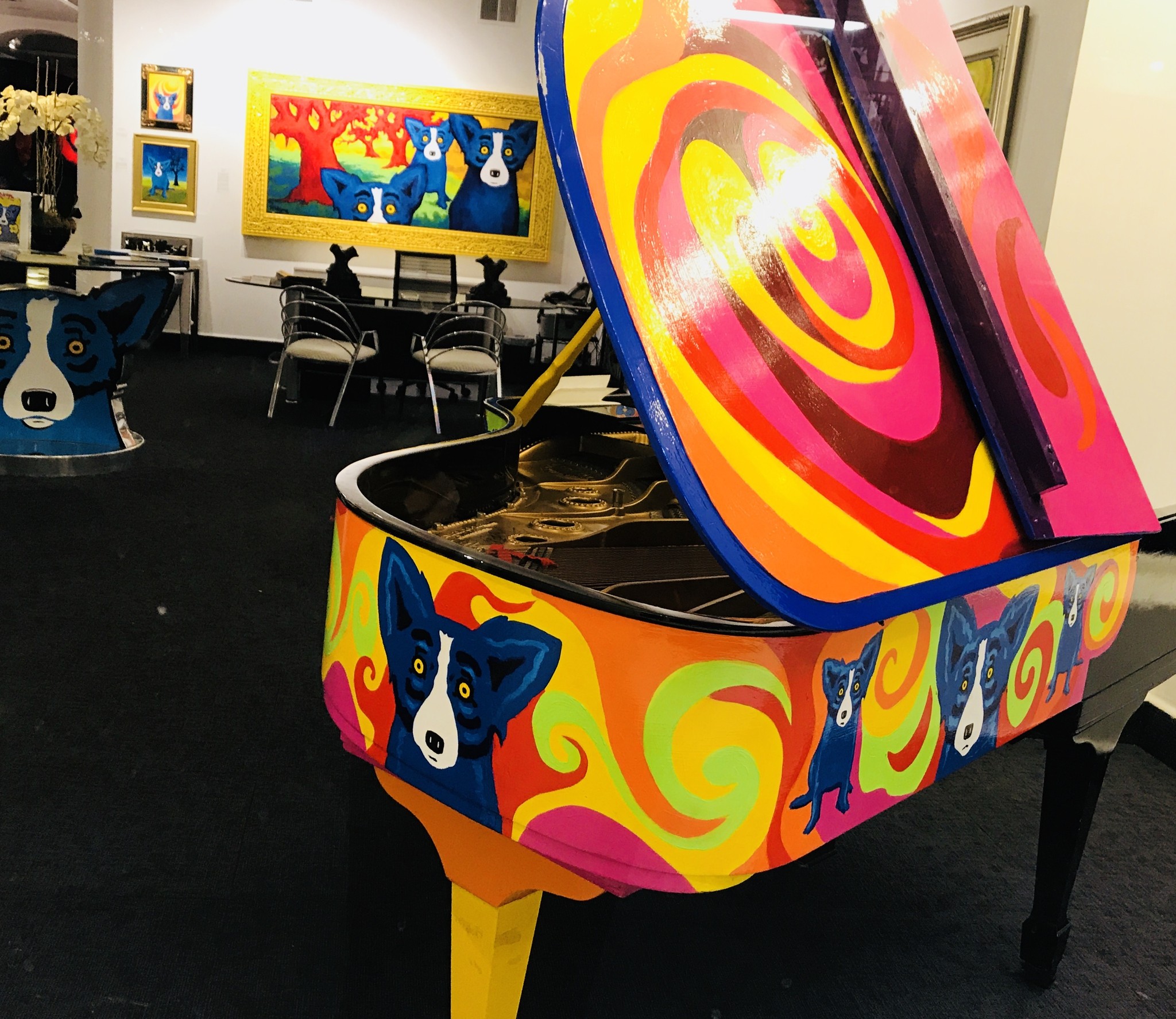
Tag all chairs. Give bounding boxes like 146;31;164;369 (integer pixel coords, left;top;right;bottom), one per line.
267;284;379;426
394;249;472;399
410;300;506;435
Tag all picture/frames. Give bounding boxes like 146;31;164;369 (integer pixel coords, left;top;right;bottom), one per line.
121;232;192;258
950;4;1030;162
241;71;557;262
141;64;193;133
0;189;31;252
132;133;199;217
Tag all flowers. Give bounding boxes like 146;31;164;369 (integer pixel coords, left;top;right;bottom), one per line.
0;57;109;214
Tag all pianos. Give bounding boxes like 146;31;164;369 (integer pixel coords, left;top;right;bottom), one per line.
321;0;1162;1019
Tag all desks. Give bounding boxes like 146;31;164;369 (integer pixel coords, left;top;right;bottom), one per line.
226;275;556;418
0;249;199;480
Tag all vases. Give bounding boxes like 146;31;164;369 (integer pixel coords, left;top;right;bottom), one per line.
32;193;79;256
